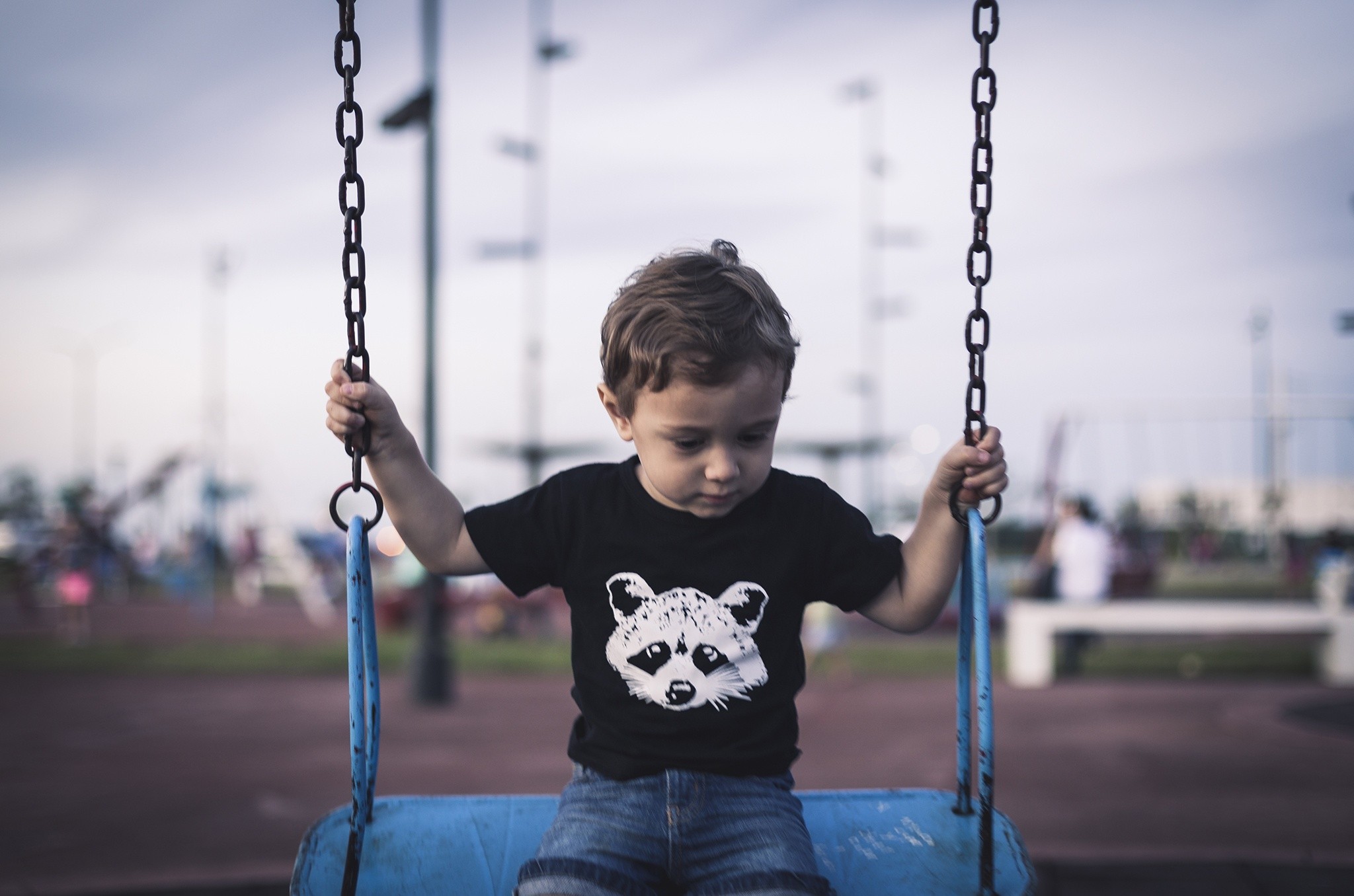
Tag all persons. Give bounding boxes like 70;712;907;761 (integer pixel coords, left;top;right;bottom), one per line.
1010;490;1150;676
325;238;1010;896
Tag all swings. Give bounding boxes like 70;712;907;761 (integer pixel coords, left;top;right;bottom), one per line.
287;1;1042;895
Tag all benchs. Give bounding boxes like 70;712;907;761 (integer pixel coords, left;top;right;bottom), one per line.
999;595;1353;691
